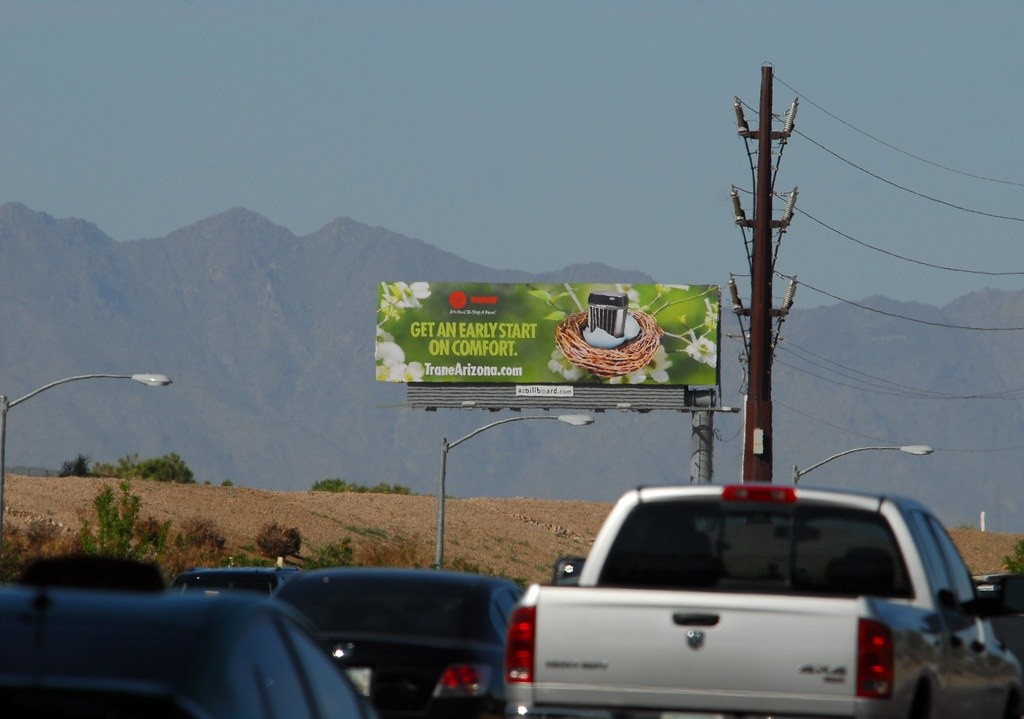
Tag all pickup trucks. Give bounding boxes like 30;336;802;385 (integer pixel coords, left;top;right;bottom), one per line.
497;484;1024;719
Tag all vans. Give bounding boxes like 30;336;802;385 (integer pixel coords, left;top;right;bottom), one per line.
172;565;299;601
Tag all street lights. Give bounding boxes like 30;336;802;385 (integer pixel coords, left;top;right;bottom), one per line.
0;372;176;580
433;413;596;576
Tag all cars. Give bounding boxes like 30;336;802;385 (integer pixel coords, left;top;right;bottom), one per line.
263;569;538;719
0;583;383;719
